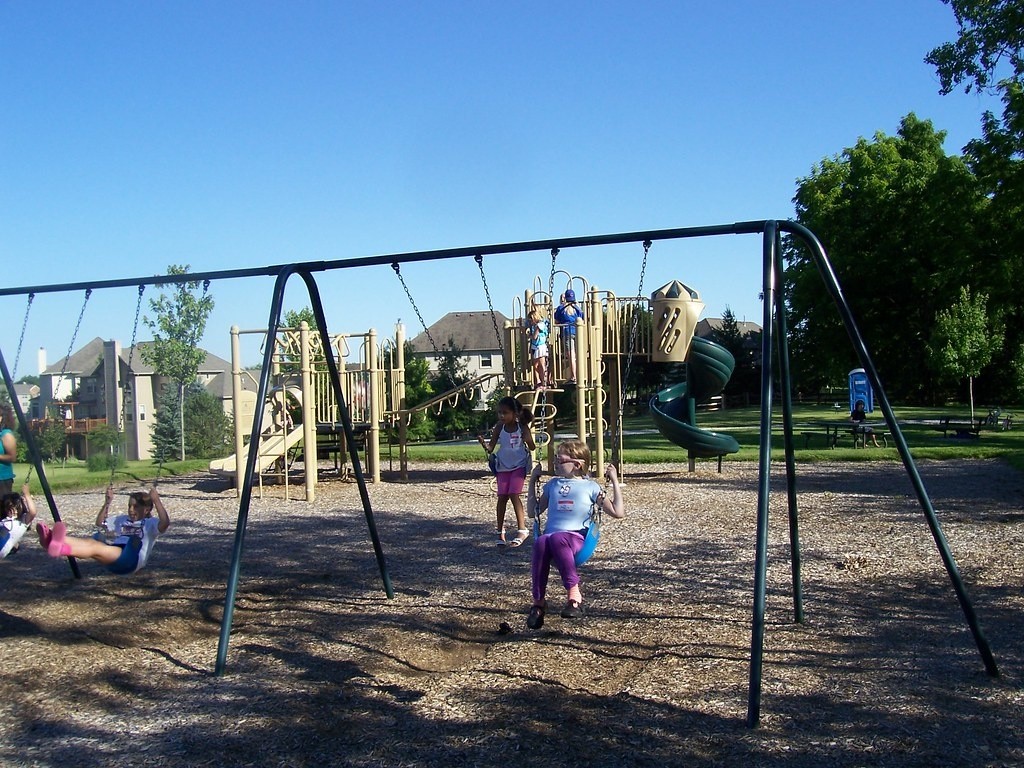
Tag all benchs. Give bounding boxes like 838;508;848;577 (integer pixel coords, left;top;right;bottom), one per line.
845;428;891;448
801;432;846;450
984;414;1013;430
931;415;988;439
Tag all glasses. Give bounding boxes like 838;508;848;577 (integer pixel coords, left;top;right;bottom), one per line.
552;455;583;470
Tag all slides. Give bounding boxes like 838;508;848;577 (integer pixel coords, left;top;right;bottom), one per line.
206;421;307;483
645;332;742;459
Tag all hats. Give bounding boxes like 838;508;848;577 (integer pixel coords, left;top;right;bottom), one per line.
565;290;575;302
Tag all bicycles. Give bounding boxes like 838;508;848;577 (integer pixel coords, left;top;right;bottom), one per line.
1001;413;1015;431
985;408;1002;428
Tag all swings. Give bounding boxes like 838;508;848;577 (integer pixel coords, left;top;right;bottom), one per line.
86;279;211;579
0;283;94;556
528;237;655;574
389;253;534;481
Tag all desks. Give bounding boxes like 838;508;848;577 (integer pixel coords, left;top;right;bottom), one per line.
818;421;887;449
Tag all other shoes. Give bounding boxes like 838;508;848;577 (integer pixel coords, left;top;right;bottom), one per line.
873;443;880;447
527;598;546;629
534;382;546;391
48;521;66;558
35;521;52;550
8;546;20;554
565;378;577;385
561;599;585;618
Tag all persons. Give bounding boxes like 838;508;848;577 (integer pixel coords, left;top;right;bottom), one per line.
0;404;21;554
36;487;170;576
849;399;881;448
993;406;1013;431
477;395;536;548
0;483;37;562
519;308;551;388
526;438;625;630
554;289;584;385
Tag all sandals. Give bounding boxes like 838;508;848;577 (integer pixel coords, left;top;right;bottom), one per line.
494;529;506;547
509;527;529;547
